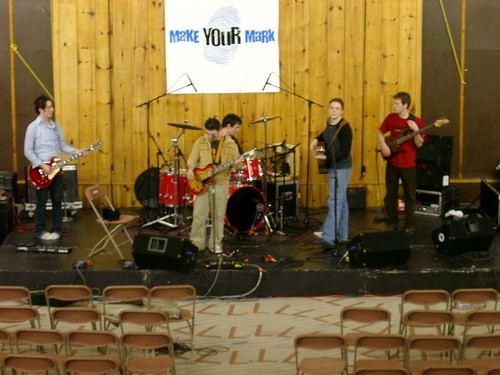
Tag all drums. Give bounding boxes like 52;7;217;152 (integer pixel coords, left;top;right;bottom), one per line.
225;184;267;233
238;152;264;181
158;166;194;207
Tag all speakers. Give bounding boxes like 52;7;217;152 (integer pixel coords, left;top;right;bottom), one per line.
415;135;453;192
131;232;199;271
432;216;495;256
346;187;366;210
25;163;77;203
347;229;411;269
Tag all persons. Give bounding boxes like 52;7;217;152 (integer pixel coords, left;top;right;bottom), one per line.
311;97;353;248
377;92;428;234
24;95;89;240
188;113;245;254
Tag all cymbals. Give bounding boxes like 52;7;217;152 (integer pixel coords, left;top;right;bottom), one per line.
168;122;203;130
249;116;281;123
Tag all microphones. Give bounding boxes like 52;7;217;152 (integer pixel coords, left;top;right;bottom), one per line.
187;74;197;92
263;73;272;91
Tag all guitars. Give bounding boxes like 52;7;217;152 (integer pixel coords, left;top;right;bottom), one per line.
187;148;257;195
380;117;450;160
27;140;103;189
314;142;329;174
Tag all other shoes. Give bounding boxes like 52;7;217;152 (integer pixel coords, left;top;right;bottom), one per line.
385;223;398;233
312;231;335;249
48;232;62;240
37;232;51;240
208;245;223;254
404;226;415;236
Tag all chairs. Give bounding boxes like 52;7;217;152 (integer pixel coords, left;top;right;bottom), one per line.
0;285;197;375
294;288;500;375
84;184;138;260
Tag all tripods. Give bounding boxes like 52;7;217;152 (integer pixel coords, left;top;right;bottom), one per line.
268;83;324;230
121;84;234;236
